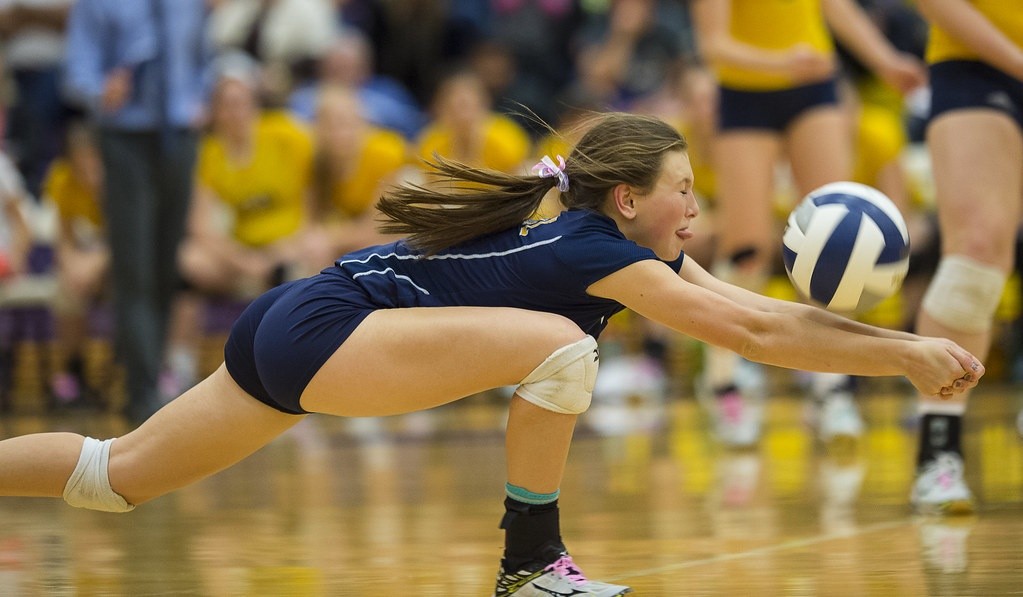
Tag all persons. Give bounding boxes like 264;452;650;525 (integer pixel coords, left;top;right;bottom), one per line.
0;97;985;597
0;0;1023;511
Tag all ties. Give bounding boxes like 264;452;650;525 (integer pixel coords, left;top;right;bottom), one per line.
145;0;170;125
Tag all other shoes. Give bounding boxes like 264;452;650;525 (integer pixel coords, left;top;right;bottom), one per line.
912;451;971;508
694;375;759;448
816;390;857;442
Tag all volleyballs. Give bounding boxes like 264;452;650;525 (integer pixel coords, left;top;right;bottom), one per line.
782;181;910;313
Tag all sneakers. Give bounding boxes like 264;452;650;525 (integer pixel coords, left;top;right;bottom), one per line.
494;542;632;596
